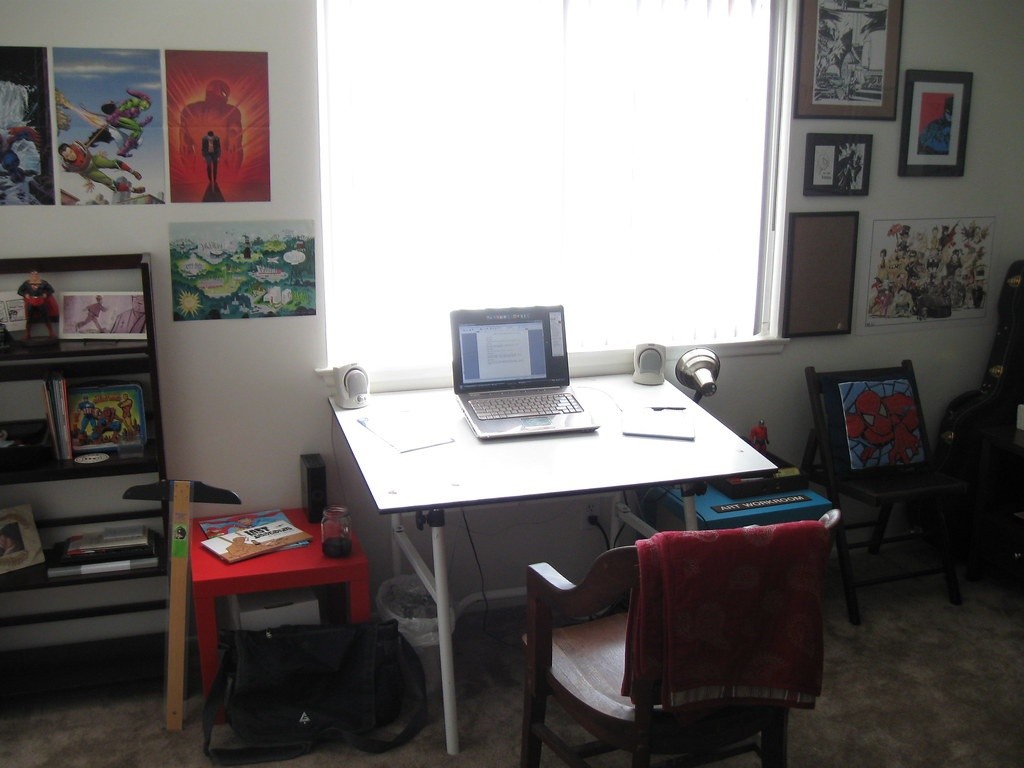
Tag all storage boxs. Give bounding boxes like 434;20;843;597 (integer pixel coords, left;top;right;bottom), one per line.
0;416;50;473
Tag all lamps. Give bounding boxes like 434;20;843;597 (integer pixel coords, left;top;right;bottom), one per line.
675;347;721;404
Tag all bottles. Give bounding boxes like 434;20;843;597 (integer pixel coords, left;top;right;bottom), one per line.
320;508;353;560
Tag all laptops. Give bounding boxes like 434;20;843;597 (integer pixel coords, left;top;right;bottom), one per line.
450;304;601;439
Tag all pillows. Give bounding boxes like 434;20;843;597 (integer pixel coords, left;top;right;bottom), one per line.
821;370;930;479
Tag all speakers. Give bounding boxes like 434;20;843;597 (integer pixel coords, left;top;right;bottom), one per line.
633;344;669;384
334;362;370;409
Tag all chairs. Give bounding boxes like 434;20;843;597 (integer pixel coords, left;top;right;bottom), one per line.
518;508;845;768
799;359;962;626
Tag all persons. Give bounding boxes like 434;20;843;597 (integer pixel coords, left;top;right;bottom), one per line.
750;419;769;451
17;270;58;340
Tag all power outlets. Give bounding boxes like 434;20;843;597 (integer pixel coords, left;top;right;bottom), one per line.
580;501;601;530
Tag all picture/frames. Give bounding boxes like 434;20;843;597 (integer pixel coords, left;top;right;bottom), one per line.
0;291;27;333
792;0;904;121
781;211;860;338
897;68;974;177
802;131;874;197
0;504;45;574
58;290;147;340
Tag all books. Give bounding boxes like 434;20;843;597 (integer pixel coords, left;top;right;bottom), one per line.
41;371;74;462
42;525;167;587
357;410;454;454
199;509;314;565
622;407;695;440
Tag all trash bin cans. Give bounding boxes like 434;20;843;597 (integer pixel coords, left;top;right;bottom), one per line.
378;575;486;701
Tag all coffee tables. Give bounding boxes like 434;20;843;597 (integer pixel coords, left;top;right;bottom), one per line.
188;504;370;725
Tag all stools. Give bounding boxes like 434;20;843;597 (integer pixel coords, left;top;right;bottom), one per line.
634;483;833;539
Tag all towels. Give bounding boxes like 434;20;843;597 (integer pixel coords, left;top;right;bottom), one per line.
620;521;829;711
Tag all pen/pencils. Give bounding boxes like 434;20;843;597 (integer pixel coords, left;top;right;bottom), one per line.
650;406;686;411
623;431;695;442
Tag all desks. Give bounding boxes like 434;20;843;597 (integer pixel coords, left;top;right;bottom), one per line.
964;423;1024;592
327;372;781;757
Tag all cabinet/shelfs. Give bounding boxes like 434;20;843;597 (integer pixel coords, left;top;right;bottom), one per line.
0;252;195;707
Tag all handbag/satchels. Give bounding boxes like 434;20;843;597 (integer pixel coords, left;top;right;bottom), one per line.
200;617;427;766
